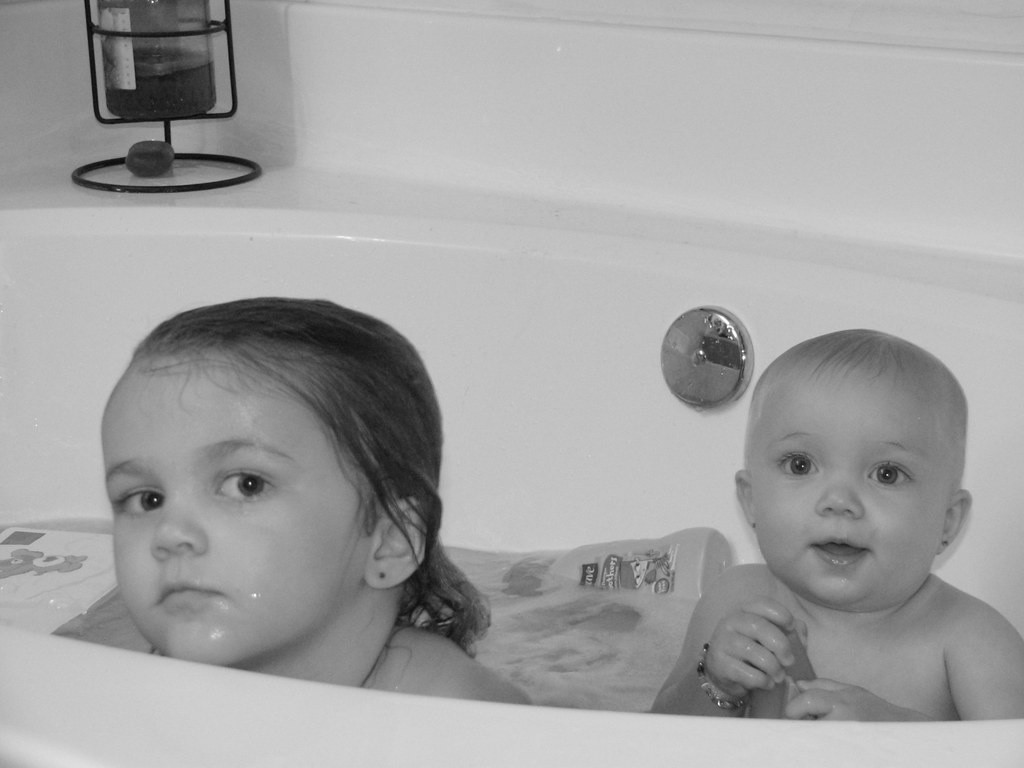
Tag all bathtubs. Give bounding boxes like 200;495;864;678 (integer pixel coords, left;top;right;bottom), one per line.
1;176;1024;768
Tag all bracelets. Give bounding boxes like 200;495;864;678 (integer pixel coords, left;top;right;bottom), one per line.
696;642;753;710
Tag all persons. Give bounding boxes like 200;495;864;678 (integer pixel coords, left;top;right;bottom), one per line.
648;328;1024;722
99;297;534;705
